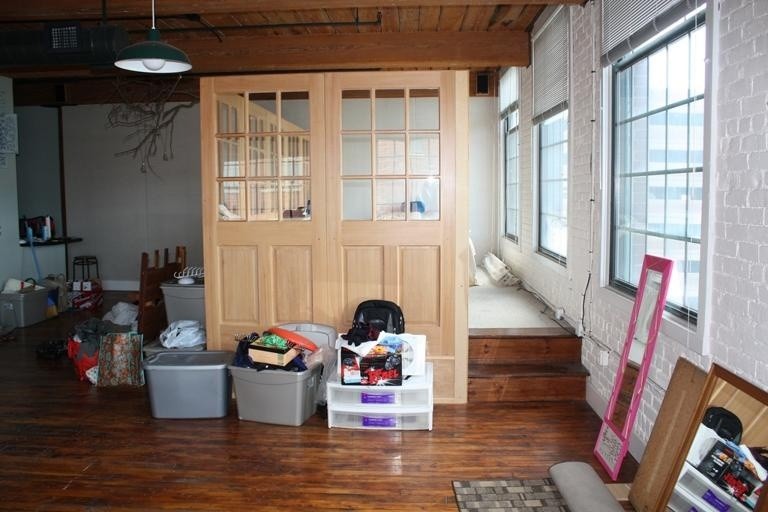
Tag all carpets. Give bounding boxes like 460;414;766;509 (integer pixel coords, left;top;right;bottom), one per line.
451;462;627;512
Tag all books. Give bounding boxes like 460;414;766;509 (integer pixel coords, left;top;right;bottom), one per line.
247;342;301;369
687;440;764;512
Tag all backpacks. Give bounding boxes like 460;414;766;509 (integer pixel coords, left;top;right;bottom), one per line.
352;300;405;335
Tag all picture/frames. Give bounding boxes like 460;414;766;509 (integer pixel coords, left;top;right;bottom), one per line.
593;417;629;481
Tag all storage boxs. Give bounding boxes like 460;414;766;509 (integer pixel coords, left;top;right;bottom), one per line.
142;323;433;431
159;277;207;328
0;287;51;328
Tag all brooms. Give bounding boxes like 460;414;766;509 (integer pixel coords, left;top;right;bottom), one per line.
23;216;57;318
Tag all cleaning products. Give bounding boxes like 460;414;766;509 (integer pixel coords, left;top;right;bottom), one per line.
46;215;50;238
42;226;47;240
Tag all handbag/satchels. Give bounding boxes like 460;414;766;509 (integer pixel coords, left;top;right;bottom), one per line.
97;330;144;388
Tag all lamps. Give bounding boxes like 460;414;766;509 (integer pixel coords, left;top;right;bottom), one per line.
113;0;193;74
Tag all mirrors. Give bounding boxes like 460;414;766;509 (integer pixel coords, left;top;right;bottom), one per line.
605;254;674;459
653;361;768;512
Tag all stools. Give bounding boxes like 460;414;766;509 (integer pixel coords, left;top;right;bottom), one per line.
73;256;99;281
